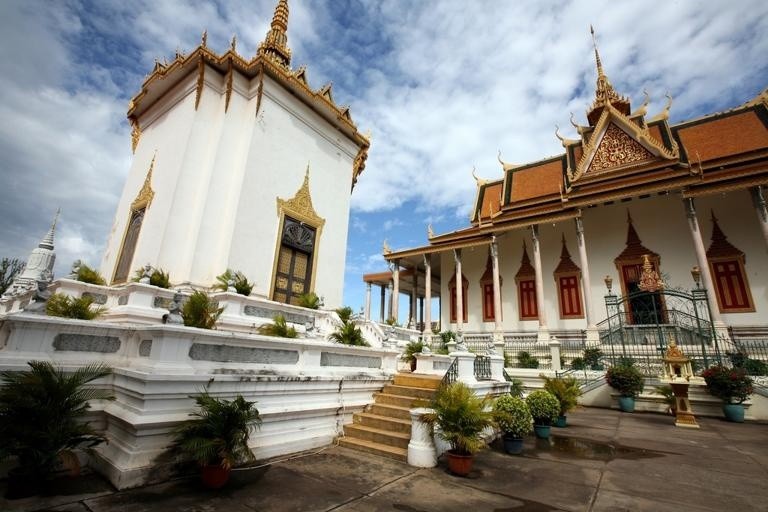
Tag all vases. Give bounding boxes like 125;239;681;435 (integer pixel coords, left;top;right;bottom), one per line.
721;404;744;424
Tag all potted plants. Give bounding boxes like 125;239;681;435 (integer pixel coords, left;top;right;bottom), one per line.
418;382;502;476
491;393;532;455
163;377;263;489
604;365;645;411
0;359;117;500
539;373;585;428
400;340;422;372
525;389;560;439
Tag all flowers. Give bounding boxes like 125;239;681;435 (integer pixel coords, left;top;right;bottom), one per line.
701;366;753;404
